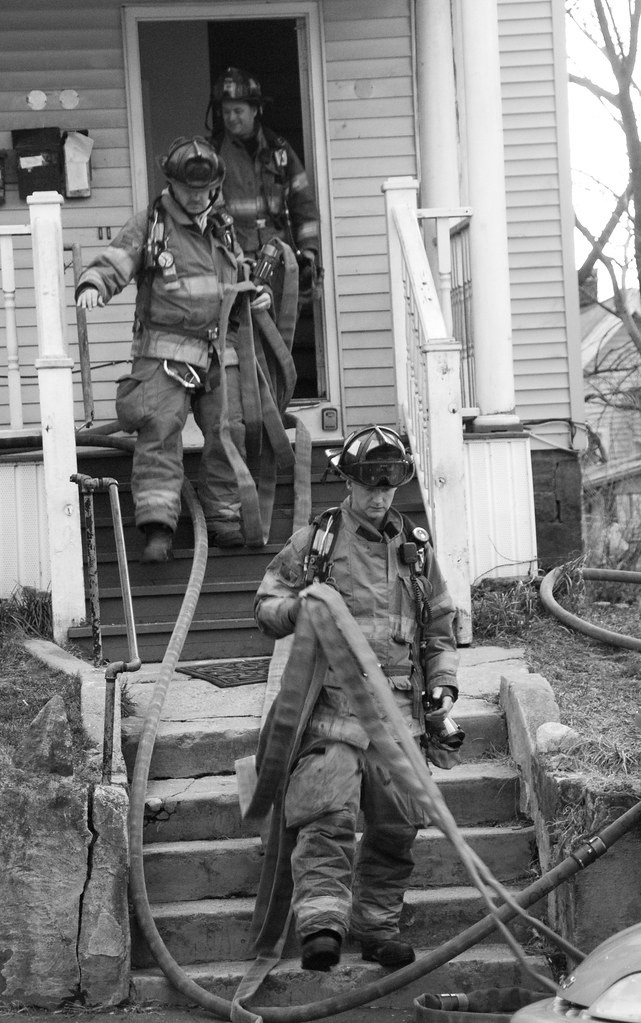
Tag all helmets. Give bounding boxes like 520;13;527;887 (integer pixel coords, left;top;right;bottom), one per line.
324;423;416;488
210;67;272;104
156;136;227;192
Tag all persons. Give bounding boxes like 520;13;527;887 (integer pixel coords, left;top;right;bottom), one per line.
254;423;460;969
213;65;320;428
75;138;272;563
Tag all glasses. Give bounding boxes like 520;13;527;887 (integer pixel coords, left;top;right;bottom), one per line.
351;461;409;486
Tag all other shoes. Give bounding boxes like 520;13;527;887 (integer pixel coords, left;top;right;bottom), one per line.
208;530;245;549
360;941;414;966
301;937;342;972
141;527;174;562
